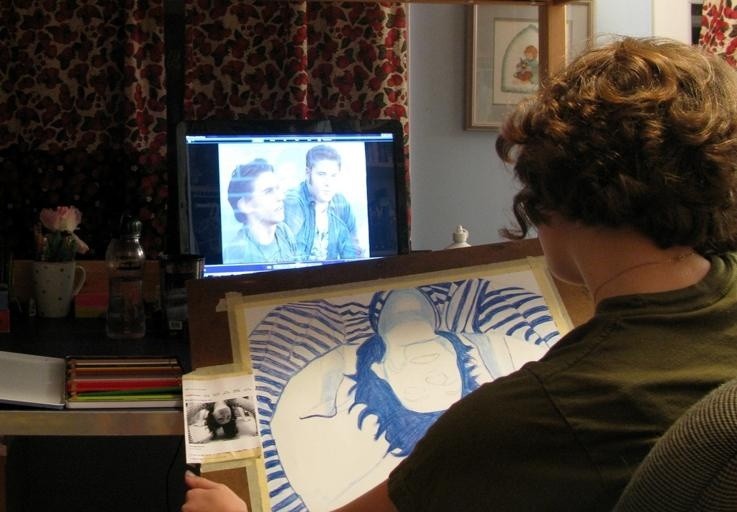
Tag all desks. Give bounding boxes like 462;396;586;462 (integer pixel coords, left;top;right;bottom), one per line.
2;316;190;450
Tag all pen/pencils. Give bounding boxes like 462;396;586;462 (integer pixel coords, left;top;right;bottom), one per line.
38;234;78;263
66;358;184;402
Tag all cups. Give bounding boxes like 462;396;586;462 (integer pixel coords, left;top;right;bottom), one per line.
32;261;86;321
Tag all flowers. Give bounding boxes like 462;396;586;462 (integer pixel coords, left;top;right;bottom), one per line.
37;206;89;261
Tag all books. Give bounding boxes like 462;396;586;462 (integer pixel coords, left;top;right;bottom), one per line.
0;350;182;410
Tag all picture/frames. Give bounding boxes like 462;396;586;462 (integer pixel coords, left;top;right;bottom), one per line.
463;2;595;133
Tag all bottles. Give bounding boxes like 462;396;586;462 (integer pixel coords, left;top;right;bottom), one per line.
446;225;475;251
107;216;147;343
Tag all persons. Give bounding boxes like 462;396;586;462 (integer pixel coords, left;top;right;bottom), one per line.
185;397;258;444
222;157;302;266
284;145;364;263
180;35;736;511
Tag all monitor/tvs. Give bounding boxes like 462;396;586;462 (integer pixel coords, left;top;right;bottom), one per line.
171;116;411;278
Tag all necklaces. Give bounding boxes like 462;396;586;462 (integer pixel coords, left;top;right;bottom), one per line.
592;246;696;304
